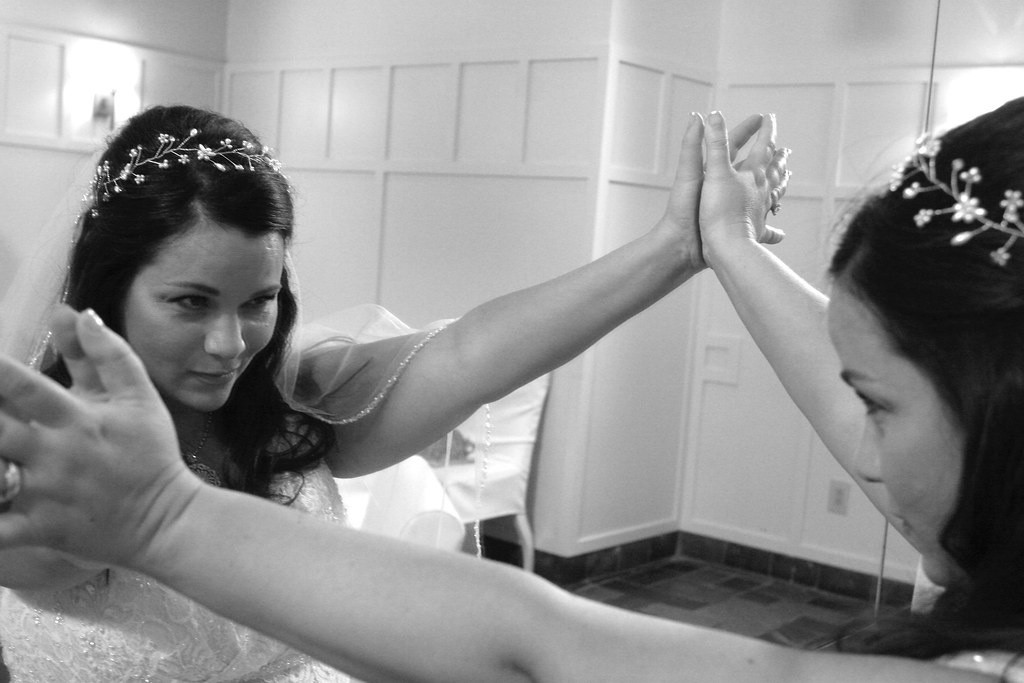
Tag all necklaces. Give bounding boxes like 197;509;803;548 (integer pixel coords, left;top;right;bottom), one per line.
179;413;214;459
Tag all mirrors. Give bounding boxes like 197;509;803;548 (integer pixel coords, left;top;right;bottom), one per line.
0;0;938;683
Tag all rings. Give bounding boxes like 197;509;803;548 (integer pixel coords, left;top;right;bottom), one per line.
0;462;22;503
772;191;781;216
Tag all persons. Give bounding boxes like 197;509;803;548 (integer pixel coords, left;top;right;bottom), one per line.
0;107;763;683
0;96;1024;683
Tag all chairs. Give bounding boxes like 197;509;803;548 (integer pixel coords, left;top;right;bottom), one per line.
412;318;556;575
